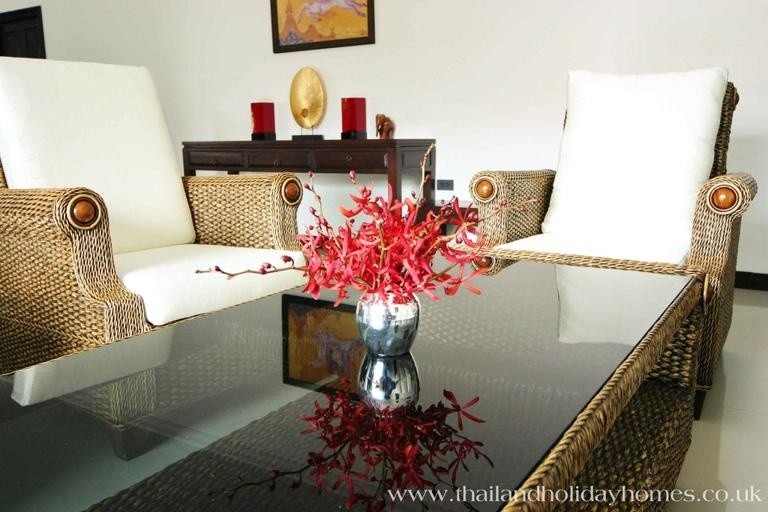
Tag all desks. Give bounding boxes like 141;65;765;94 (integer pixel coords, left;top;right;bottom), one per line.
5;247;718;512
182;140;435;222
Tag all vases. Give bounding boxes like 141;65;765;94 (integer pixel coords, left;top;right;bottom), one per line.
359;354;420;415
356;288;420;358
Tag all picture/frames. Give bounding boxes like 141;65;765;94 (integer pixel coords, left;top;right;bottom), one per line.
269;0;376;55
281;295;366;400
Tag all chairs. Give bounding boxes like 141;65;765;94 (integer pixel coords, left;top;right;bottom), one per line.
3;53;316;462
468;80;757;420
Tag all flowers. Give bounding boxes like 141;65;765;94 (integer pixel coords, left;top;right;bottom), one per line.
195;143;542;310
205;377;496;512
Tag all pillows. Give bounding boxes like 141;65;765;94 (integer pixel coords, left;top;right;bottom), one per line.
541;67;729;257
